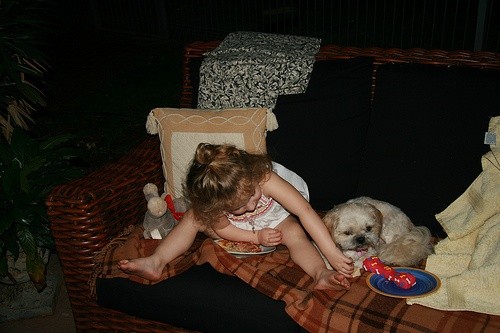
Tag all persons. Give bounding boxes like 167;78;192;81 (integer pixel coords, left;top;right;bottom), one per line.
117;143;355;292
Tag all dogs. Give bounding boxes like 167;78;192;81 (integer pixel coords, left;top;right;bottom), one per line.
321;197;432;266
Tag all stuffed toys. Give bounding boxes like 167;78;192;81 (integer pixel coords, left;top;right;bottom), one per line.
142;183;188;240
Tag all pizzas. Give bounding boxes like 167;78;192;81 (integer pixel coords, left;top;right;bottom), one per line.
219;239;262;252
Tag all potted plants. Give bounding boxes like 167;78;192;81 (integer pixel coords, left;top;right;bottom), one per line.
0;0;104;322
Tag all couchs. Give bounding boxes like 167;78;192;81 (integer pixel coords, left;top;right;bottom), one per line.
51;39;500;333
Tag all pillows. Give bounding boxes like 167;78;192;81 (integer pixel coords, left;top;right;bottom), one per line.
146;105;279;200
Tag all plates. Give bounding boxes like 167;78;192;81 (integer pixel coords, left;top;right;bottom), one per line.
214;239;276;254
366;267;442;298
